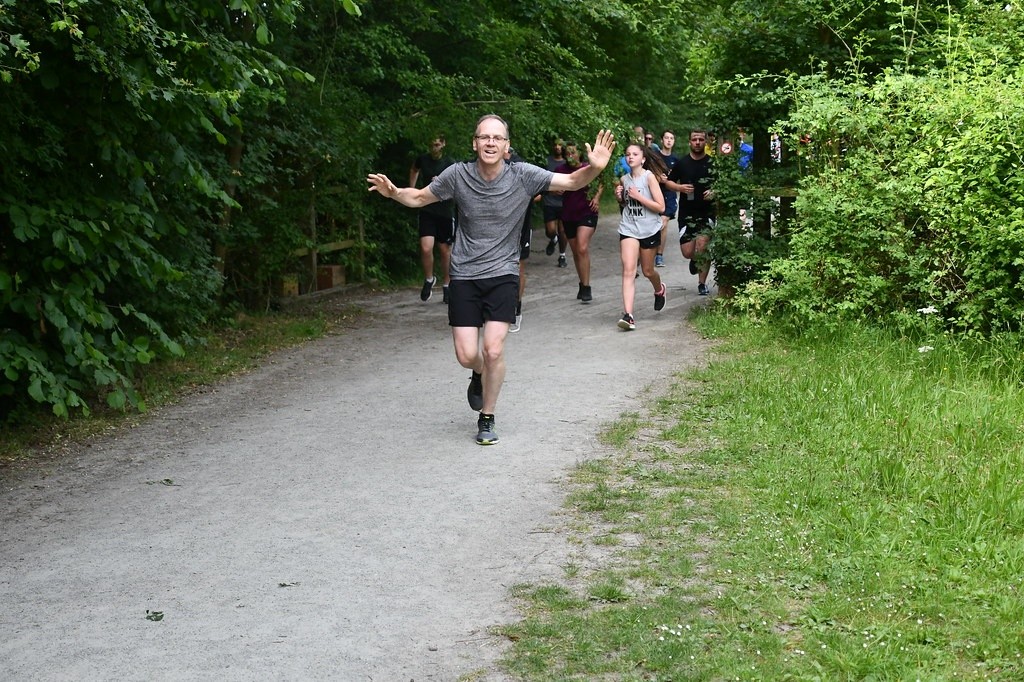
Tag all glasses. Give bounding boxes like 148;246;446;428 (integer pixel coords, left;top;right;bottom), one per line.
475;135;508;143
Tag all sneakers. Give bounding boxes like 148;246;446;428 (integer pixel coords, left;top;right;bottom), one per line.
654;283;666;311
443;286;449;303
655;255;665;267
509;315;523;332
546;234;559;255
558;256;568;268
467;370;483;410
698;283;709;295
689;259;698;275
577;284;591;301
477;412;500;444
421;276;436;301
617;312;636;330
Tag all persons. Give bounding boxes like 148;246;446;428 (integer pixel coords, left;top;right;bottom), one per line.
410;133;459;304
502;125;719;333
366;115;616;445
